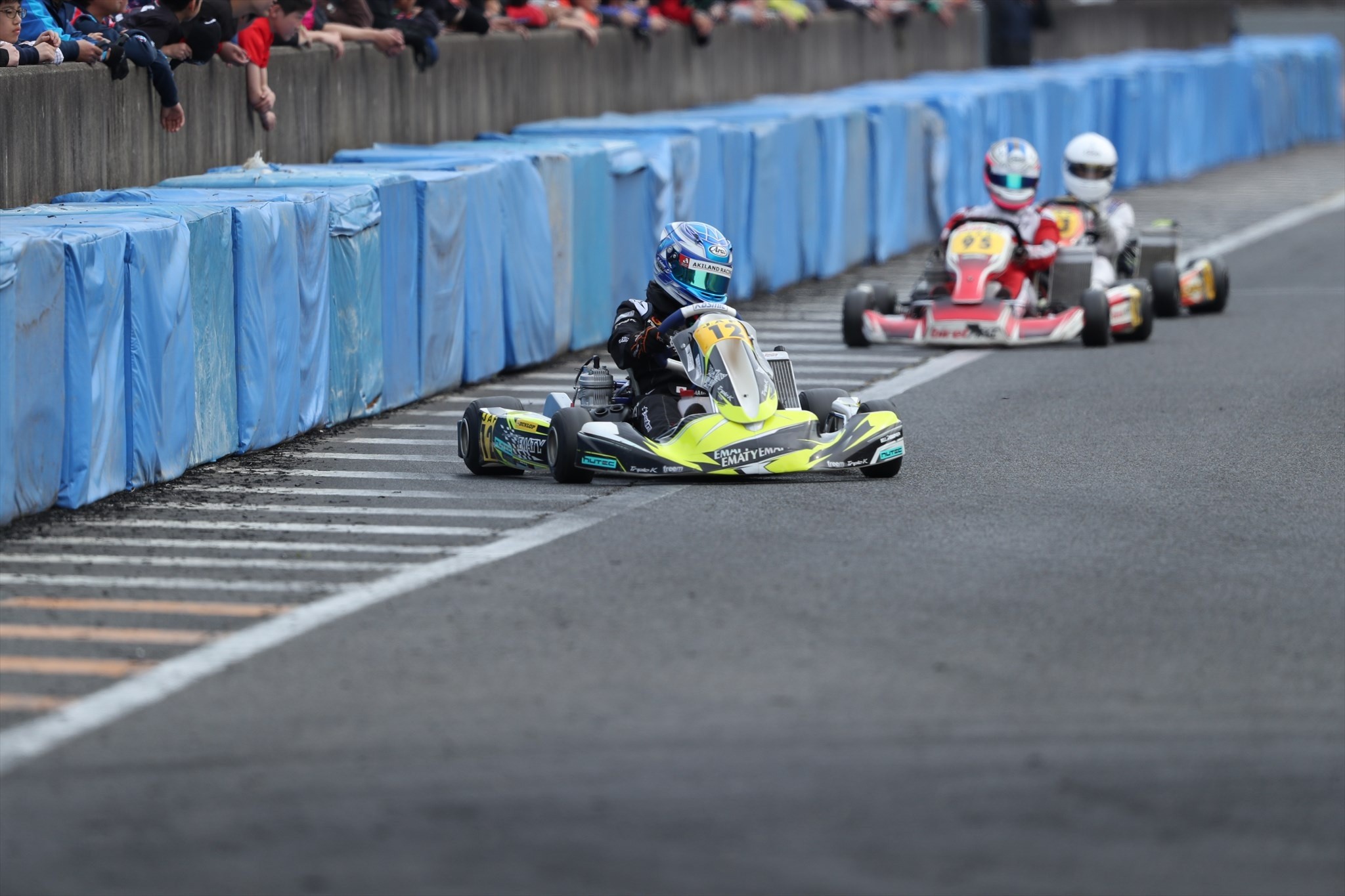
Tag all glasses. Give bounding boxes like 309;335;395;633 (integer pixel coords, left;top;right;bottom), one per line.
0;8;27;19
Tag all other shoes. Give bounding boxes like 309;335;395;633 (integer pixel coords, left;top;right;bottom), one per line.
995;288;1012;300
669;403;707;438
931;286;948;299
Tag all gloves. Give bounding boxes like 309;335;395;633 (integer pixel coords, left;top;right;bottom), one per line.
1012;245;1028;266
631;326;670;361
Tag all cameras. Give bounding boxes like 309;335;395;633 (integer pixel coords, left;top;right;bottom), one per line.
95;39;109;52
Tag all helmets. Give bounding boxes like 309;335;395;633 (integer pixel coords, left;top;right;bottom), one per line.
984;137;1040;210
1062;131;1119;203
654;220;734;306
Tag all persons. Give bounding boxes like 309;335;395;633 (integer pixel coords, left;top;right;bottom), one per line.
0;0;1055;132
607;221;784;441
930;137;1060;317
1060;132;1135;292
